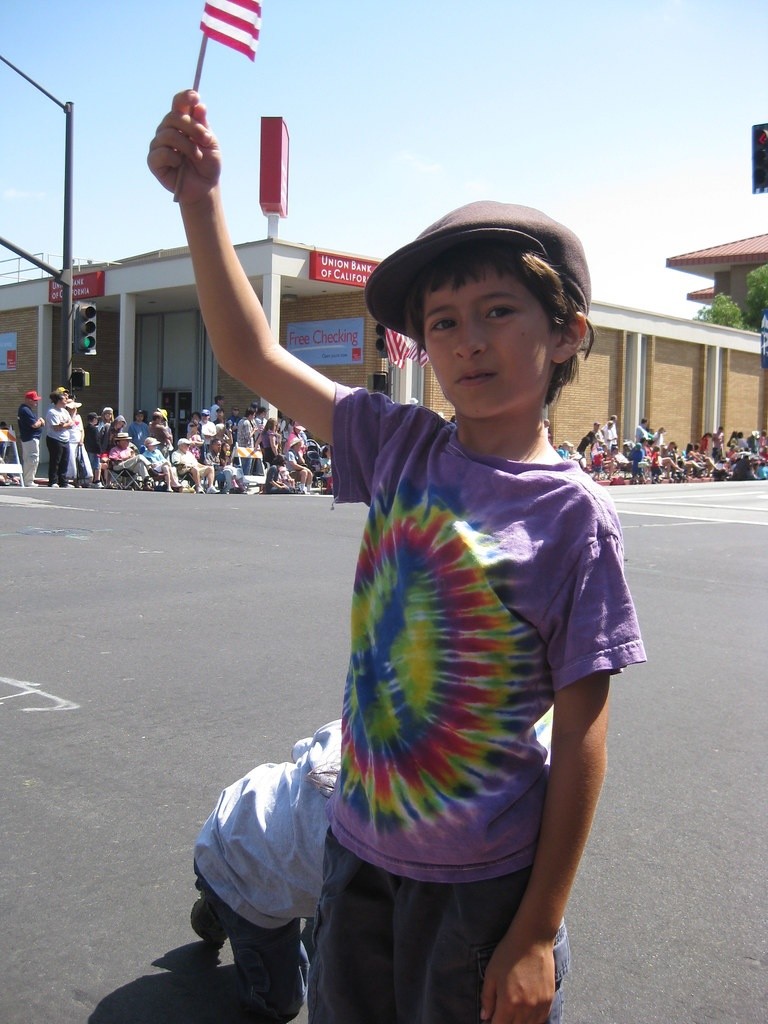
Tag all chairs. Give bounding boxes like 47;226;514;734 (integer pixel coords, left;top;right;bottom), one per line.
108;444;217;494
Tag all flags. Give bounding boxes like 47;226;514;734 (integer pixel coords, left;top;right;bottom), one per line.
198;0;262;63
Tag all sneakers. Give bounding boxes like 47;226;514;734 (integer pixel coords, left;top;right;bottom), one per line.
94;482;104;488
196;486;204;494
65;484;74;488
206;487;220;494
26;482;39;487
51;483;60;488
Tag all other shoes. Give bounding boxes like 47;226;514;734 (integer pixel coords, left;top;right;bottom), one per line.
142;485;155;491
171;480;182;488
105;484;117;489
153;472;167;480
166;488;173;492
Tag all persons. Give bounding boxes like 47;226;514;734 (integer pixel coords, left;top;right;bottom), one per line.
46;387;334;495
191;719;342;1024
543;415;768;486
0;421;9;458
17;391;45;488
147;89;646;1024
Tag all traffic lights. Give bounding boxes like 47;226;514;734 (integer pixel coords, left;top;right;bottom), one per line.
72;371;89;386
74;300;97;355
752;123;768;194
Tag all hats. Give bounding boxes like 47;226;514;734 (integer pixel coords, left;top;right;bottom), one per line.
134;409;143;416
593;422;600;426
634;443;641;448
143;436;161;446
177;438;193;446
191;435;205;444
290;437;301;448
55;387;69;394
201;409;211;416
66;399;82;408
563;441;572;446
362;199;593;344
25;391;42;401
157;408;167;421
113;432;133;441
